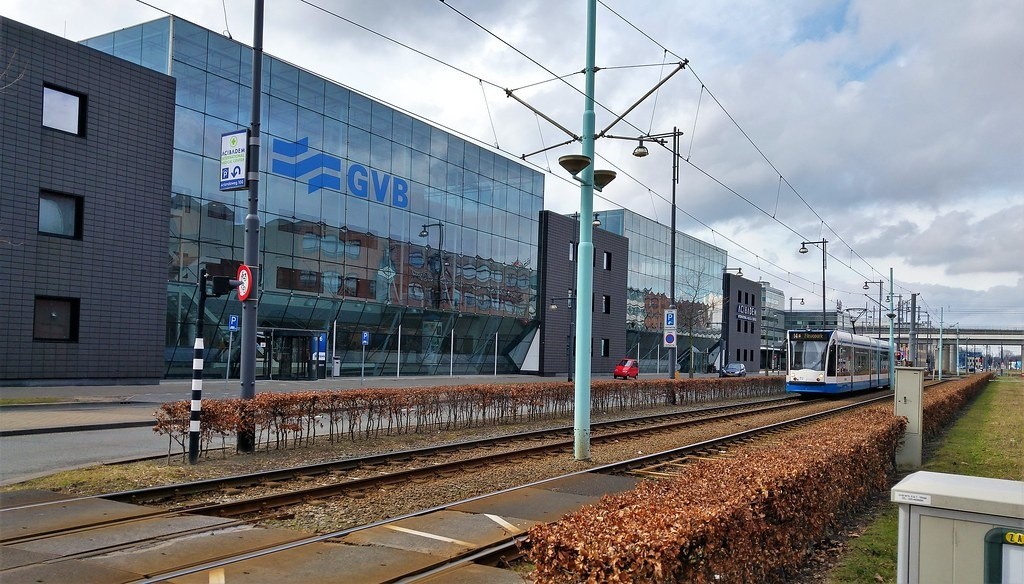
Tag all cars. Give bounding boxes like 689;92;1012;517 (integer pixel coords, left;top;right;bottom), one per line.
721;361;746;377
614;359;638;380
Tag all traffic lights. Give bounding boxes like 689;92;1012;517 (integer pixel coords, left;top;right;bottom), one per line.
212;276;243;296
897;352;901;360
774;355;776;359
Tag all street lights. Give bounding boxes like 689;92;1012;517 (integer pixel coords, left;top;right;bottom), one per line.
419;219;443;308
906;307;921;367
632;126;684;406
922;321;932;370
885;296;903;365
790;297;804;329
548;211;602;382
863;280;884;340
705;266;743;340
862;321;871;334
800;238;828;330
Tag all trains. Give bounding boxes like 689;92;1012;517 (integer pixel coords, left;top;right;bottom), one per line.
785;308;897;396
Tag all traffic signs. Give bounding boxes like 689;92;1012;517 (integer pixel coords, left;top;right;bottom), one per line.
219;129;246;191
